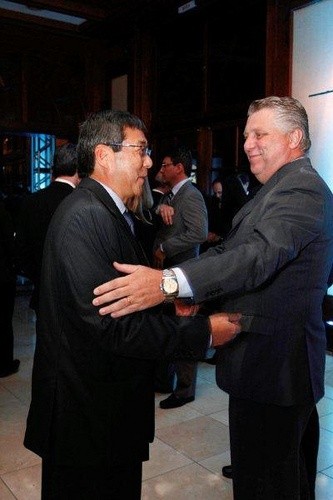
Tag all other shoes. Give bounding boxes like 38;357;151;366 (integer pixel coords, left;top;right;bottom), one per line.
0;359;20;377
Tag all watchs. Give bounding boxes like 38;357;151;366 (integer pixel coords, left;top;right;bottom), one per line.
160;268;179;304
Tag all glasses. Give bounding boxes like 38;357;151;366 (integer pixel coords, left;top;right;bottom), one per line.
103;142;151;155
162;163;177;167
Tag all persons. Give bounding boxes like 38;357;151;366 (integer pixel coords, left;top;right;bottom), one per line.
150;149;208;409
207;176;240;248
23;110;243;500
25;142;85;310
0;197;24;378
91;95;333;500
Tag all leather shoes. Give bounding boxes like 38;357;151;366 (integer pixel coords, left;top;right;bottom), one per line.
160;394;195;409
